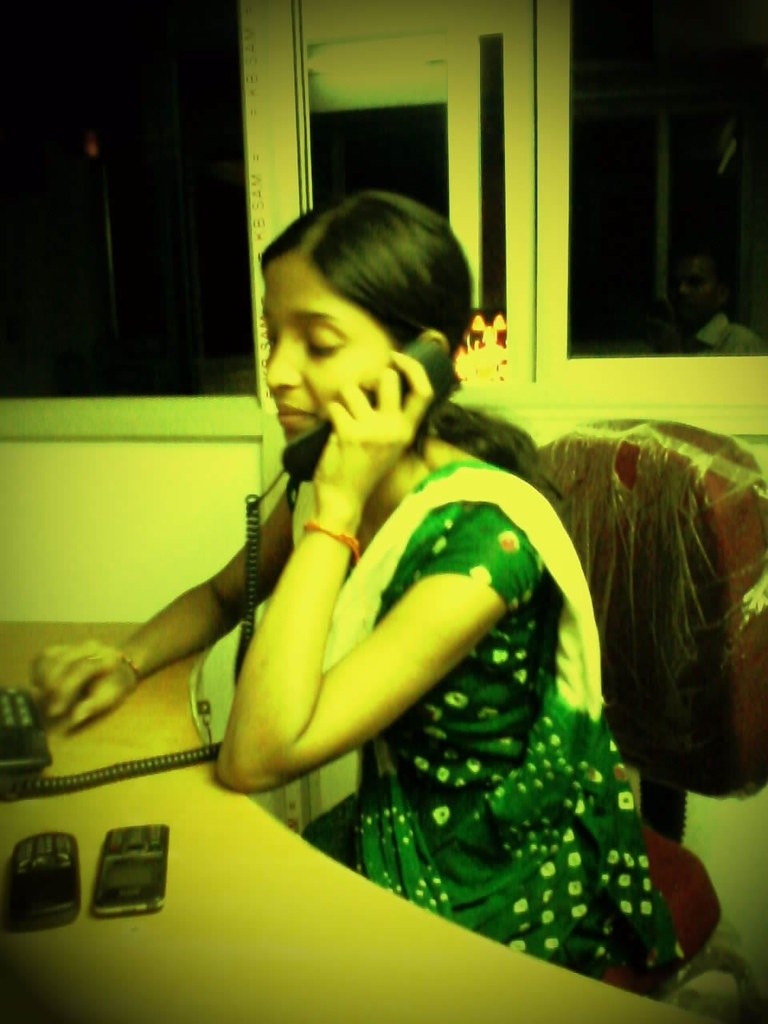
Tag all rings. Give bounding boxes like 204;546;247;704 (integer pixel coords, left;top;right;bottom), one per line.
88;655;102;663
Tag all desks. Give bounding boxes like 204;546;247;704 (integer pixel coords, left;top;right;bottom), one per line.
0;618;725;1024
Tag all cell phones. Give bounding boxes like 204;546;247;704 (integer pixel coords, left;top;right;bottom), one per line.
92;825;171;918
3;831;83;931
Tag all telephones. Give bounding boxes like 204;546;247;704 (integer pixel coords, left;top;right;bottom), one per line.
281;337;459;481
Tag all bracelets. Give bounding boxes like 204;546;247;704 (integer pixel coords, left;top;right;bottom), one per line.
122;654;140;678
302;520;361;566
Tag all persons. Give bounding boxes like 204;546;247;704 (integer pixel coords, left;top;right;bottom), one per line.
26;180;689;993
654;241;768;355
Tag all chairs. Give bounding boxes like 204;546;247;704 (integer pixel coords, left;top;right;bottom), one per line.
539;410;768;989
